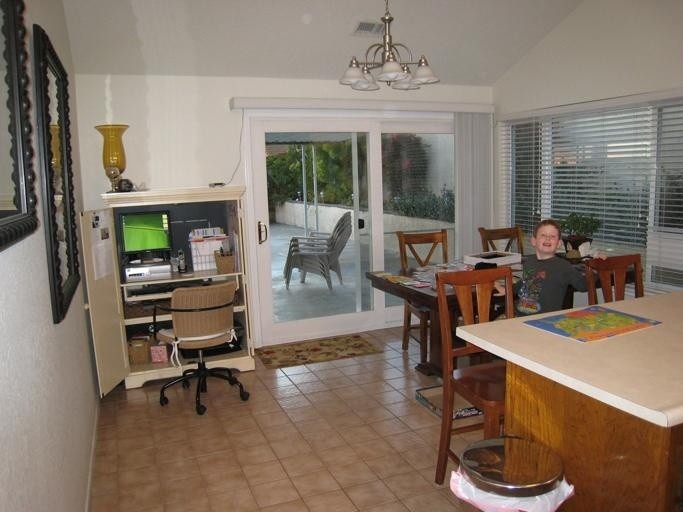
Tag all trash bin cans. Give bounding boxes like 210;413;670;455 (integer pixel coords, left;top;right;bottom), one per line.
459;435;565;512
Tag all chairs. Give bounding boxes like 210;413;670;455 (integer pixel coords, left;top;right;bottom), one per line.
282;211;352;290
151;279;250;416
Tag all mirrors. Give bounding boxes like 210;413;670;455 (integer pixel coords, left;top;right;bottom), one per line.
0;0;80;326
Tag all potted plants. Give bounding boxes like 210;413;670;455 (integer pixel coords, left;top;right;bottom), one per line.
557;212;602;240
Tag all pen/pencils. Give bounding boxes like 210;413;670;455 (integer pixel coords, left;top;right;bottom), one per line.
220;247;232;256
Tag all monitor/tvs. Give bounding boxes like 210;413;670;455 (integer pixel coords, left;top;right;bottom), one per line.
118;210;174;264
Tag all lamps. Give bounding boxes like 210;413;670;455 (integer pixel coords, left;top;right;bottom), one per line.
338;1;440;92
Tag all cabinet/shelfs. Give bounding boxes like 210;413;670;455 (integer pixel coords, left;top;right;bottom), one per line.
77;183;256;399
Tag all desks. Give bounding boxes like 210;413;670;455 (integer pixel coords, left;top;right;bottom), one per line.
455;289;682;512
364;252;644;379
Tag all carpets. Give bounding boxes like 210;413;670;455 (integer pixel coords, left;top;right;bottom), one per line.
253;333;383;370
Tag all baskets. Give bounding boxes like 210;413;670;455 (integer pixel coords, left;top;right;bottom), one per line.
189;228;230;271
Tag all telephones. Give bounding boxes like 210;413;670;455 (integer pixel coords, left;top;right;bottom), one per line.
178;249;187;273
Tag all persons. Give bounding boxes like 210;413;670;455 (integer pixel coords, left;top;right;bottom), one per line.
493;219;608;320
